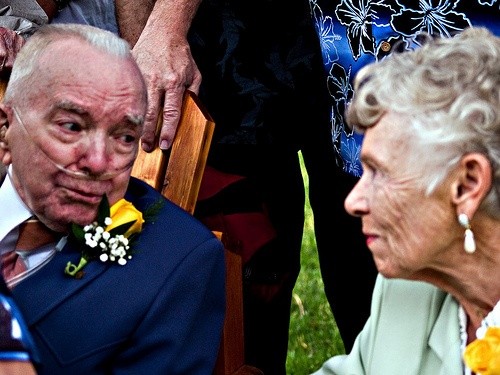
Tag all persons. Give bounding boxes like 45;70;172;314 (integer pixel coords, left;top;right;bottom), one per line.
302;26;500;375
310;0;500;179
1;1;379;375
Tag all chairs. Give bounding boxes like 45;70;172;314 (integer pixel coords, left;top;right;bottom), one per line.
127;85;245;375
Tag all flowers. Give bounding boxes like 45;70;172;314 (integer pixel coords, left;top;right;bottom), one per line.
462;327;500;375
63;192;164;276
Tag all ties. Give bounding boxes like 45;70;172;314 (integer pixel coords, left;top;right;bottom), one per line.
0;219;59;281
